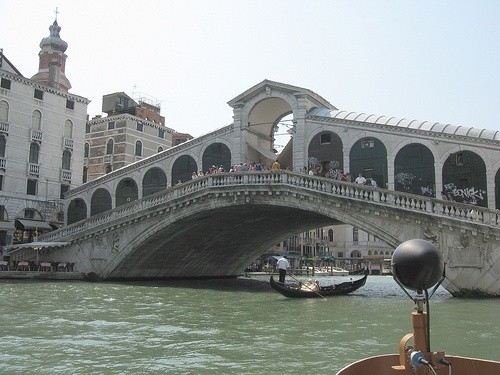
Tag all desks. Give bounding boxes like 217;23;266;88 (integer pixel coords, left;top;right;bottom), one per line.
58;262;67;267
40;262;51;266
18;262;29;265
0;261;9;265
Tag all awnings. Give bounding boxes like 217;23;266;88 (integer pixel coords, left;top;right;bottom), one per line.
277;253;300;259
50;223;64;229
15;218;53;231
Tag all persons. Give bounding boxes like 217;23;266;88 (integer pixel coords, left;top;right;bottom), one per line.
354;172;366;199
276;255;289;283
176;179;183;196
192;161;265;191
300;166;351;196
271;160;280;183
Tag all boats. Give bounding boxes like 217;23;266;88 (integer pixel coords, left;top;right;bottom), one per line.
269;267;367;299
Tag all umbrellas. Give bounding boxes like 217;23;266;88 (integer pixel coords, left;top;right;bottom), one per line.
3;241;71;265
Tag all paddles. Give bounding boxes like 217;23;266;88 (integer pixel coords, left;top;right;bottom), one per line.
285;270;326;299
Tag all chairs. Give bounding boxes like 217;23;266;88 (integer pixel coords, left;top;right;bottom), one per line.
0;261;75;272
